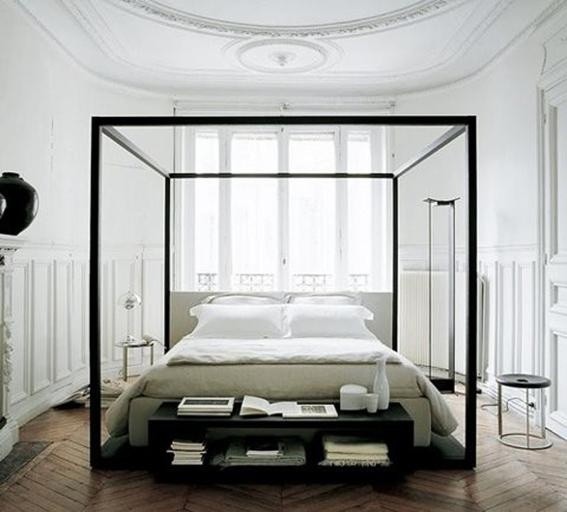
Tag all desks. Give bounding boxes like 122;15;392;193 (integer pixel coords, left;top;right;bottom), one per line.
114;338;156;383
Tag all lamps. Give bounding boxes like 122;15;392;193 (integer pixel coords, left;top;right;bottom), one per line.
118;291;142;347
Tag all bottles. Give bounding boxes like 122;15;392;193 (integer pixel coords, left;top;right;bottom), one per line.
372;357;391;414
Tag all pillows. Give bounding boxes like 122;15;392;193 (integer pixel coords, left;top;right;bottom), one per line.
186;293;378;343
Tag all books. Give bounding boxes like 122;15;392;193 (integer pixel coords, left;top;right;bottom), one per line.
166;437;209;466
177;394;339;418
246;439;284;457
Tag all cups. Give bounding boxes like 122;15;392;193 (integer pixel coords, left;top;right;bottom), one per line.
365;394;379;413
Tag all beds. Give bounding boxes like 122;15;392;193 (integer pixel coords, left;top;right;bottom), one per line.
98;288;467;476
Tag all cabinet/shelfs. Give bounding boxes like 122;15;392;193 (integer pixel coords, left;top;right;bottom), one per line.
145;403;415;494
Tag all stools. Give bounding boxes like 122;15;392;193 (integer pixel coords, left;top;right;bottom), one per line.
493;372;553;451
